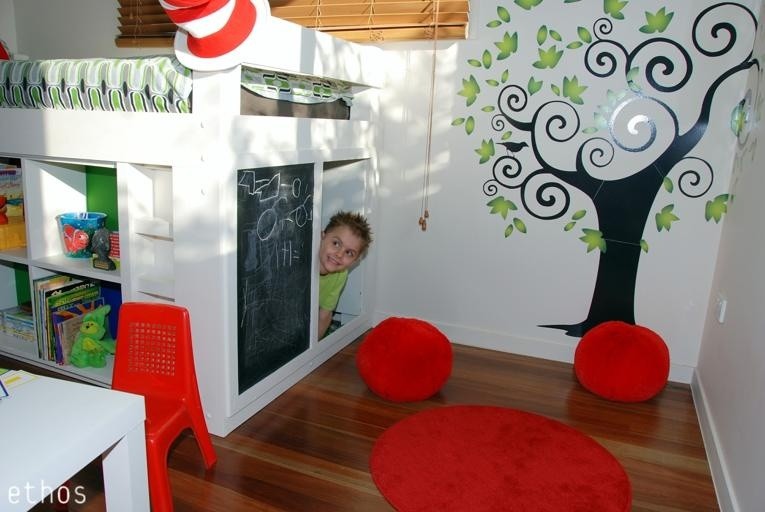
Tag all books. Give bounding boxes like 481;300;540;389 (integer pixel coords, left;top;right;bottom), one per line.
1;160;25;226
0;301;35;343
31;272;107;365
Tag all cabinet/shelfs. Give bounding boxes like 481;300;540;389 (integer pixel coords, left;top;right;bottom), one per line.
0;151;175;389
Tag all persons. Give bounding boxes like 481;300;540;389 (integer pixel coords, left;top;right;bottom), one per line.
317;209;373;343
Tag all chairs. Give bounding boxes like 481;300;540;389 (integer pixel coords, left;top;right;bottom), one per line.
111;302;219;512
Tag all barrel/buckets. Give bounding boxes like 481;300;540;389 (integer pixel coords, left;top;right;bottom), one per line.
58;214;110;261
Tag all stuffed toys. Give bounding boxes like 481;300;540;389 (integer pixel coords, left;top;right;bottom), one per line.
69;304;118;369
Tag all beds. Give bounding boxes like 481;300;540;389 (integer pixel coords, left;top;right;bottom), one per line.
0;17;385;439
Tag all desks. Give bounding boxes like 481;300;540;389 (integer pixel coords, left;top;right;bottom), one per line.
0;368;150;512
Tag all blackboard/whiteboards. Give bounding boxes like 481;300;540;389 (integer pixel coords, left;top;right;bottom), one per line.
237;163;314;395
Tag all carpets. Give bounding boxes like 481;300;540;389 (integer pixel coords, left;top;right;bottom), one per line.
369;403;634;512
